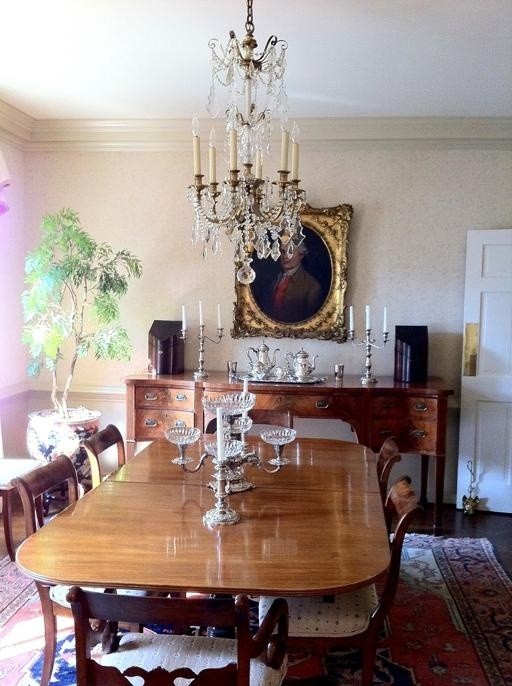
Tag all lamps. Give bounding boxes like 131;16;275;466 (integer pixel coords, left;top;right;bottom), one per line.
187;1;307;284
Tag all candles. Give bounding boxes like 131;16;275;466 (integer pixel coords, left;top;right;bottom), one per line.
216;406;226;462
244;377;249;418
350;305;388;333
182;300;224;330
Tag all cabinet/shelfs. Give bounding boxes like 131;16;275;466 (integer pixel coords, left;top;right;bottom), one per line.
126;368;453;535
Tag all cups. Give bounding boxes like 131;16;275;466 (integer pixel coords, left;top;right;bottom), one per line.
275;367;284;379
334;360;344;380
227;360;238;378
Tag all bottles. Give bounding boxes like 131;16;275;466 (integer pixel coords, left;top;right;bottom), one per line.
252;361;267;379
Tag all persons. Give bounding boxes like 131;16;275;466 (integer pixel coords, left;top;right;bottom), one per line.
260;232;324;322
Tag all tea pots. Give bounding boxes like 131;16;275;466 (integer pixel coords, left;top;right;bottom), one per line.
245;338;281;380
285;345;319;381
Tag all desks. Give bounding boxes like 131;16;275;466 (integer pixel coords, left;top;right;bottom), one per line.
15;433;390;686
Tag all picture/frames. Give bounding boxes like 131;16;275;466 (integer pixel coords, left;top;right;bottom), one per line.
230;202;353;344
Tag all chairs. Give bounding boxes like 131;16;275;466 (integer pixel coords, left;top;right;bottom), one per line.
377;434;401;497
83;423;132;488
255;475;421;686
0;456;43;565
66;586;288;686
10;453;119;686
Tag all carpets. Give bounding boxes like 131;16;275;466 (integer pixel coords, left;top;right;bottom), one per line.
1;531;510;685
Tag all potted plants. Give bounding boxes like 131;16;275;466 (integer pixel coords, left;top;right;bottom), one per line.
22;208;143;477
